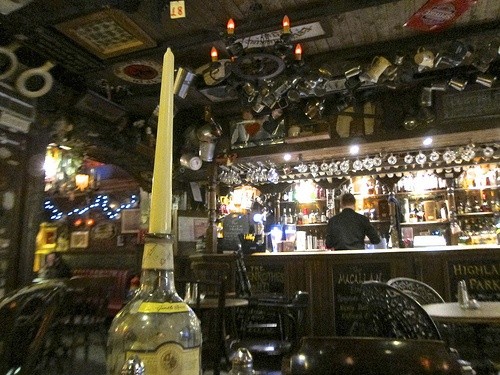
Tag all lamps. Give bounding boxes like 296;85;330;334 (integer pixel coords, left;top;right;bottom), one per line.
75;163;90;192
210;2;304;98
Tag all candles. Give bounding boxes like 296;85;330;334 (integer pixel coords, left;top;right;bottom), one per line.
146;45;176;233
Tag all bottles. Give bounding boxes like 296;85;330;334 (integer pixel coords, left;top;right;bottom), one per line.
306;227;327;248
458;194;490;213
104;233;204;375
283;184;326;202
227;346;256;375
409;204;425;222
281;209;326;225
458;279;470;308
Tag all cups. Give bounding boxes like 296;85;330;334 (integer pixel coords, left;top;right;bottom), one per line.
394;51;405;66
252;102;265;113
415;47;434;70
448;76;468;92
198;142;216;162
342;66;363;81
419;86;433;107
432;80;447;92
475;74;495;89
366;55;392;83
303;101;319;119
179;151;202;171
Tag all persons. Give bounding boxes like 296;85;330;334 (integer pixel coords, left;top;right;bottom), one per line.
38;252;71;280
325;193;383;251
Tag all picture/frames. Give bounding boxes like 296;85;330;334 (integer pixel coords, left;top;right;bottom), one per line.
120;209;142;234
69;232;88;250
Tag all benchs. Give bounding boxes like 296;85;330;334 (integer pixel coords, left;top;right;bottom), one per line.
70;265;127;316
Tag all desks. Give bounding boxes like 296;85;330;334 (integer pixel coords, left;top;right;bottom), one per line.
187;297;249;350
424;301;500;375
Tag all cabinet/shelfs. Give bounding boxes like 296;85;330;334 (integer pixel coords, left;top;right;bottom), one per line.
298;184;500;228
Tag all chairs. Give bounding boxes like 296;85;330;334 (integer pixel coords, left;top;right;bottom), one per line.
0;247;500;375
